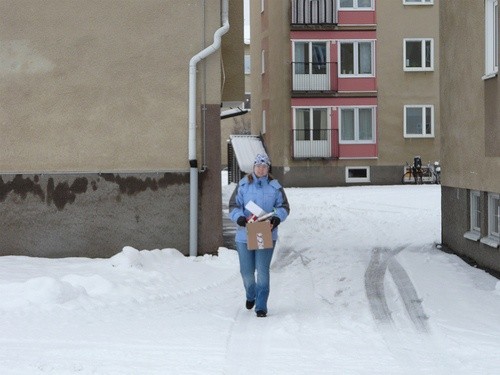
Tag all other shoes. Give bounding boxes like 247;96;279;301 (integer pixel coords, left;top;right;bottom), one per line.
257;311;266;317
246;300;255;309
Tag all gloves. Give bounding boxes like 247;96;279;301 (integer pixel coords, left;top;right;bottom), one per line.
269;216;280;230
237;216;246;227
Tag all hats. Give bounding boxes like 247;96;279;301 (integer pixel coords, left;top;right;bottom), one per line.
254;153;271;167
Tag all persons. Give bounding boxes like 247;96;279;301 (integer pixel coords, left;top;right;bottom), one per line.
228;153;292;318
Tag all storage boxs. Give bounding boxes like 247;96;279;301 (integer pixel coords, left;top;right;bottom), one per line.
246;221;274;252
